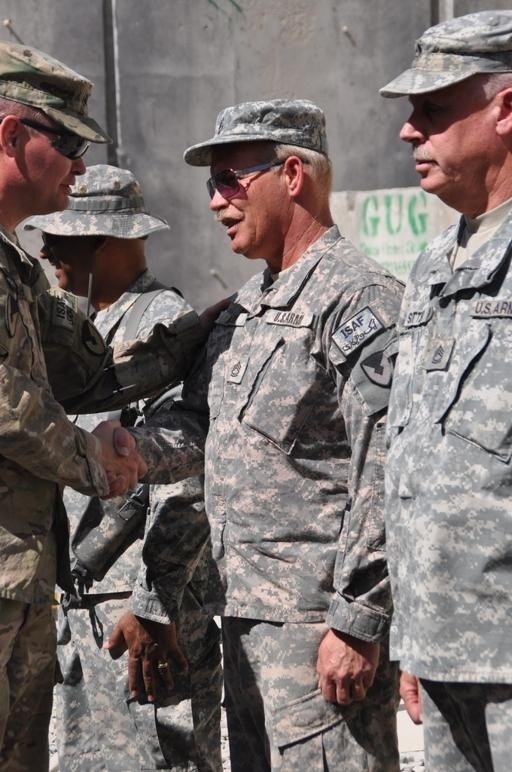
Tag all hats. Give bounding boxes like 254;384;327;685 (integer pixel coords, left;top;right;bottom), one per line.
183;98;328;166
0;42;114;144
23;164;172;240
378;9;512;97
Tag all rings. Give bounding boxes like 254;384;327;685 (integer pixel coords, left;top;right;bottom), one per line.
158;661;169;669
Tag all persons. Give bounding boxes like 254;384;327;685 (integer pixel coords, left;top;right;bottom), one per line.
23;162;222;771
380;10;512;771
2;42;234;771
89;95;416;769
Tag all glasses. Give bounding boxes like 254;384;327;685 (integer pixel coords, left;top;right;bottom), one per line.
206;158;311;199
0;117;92;160
